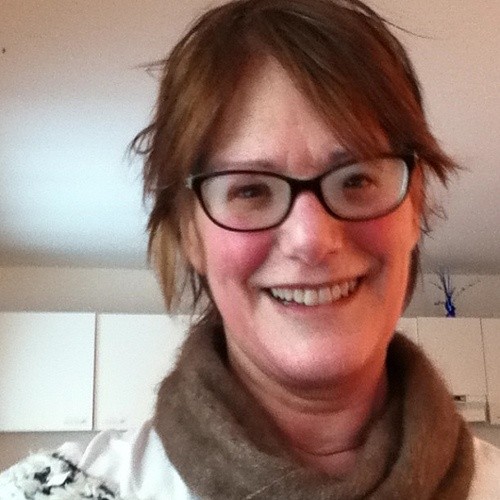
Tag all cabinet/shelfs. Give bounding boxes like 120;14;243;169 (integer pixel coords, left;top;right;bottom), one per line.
0;311;500;432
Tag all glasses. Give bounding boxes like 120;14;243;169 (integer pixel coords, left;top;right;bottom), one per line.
174;147;423;233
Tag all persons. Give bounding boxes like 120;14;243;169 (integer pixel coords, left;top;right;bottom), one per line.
0;0;500;500
